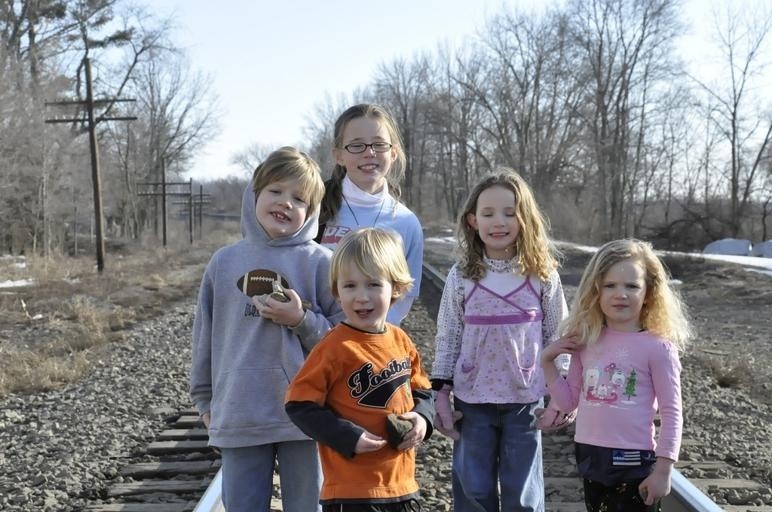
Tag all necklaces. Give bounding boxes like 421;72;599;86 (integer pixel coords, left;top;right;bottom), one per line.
341;192;387;229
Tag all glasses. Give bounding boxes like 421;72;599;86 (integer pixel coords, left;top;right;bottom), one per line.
340;140;393;154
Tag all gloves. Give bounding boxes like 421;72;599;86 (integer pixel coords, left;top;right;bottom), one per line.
533;394;579;433
428;380;464;441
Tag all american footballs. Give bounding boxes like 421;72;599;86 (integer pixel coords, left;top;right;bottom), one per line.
236;269;289;297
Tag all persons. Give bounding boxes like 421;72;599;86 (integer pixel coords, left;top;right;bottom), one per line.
281;222;440;511
309;100;425;512
186;143;350;511
536;234;693;511
423;164;576;512
